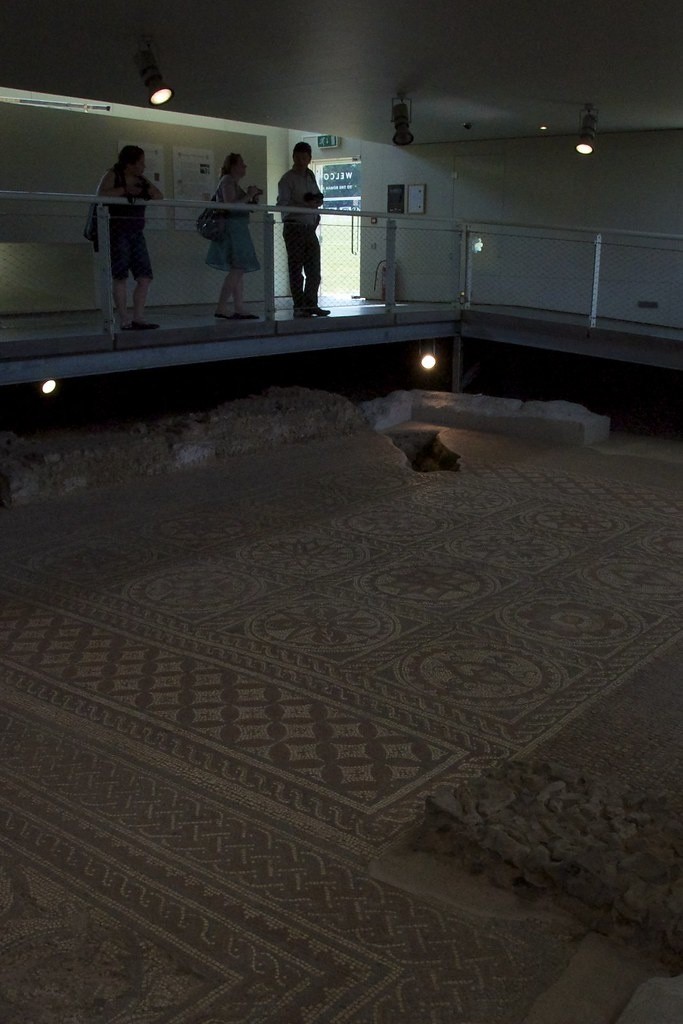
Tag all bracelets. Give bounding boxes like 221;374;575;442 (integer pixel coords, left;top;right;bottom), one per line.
122;187;129;196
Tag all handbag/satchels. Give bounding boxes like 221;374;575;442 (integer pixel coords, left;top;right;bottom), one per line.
197;198;230;243
84;204;99;240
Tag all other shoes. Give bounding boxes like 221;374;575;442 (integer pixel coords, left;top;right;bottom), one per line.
122;321;156;331
214;311;259;321
291;305;332;319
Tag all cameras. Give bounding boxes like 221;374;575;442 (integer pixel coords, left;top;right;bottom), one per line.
134;182;152;202
303;192;324;204
249;186;263;195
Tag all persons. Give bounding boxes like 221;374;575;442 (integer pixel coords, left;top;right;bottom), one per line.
276;142;330;319
205;151;262;320
97;145;163;331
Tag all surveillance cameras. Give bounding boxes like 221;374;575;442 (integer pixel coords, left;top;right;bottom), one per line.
464;123;472;130
